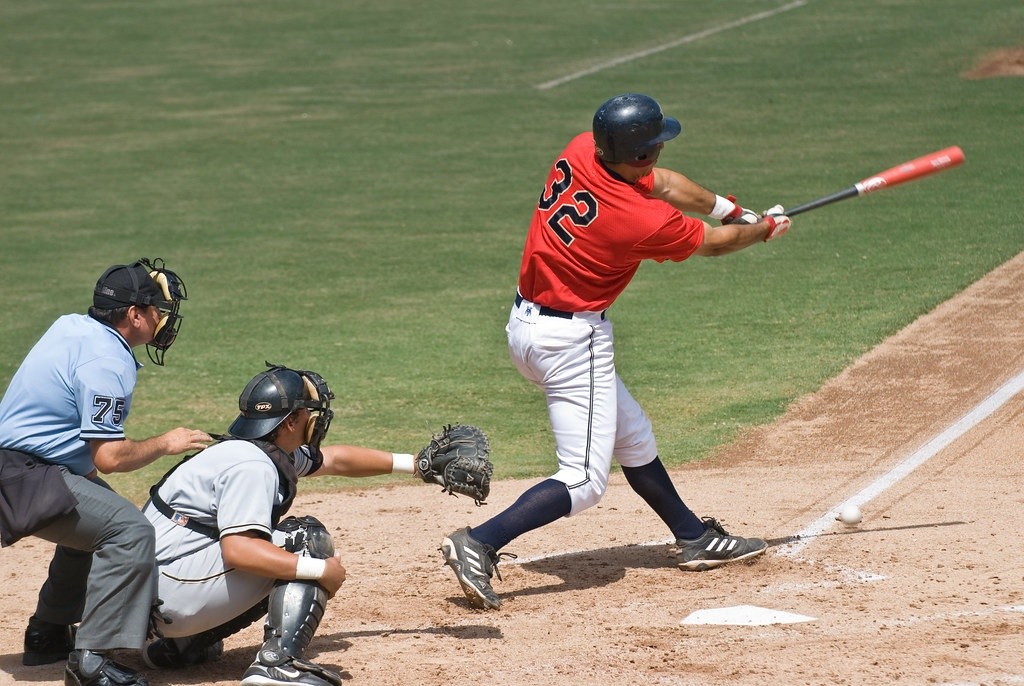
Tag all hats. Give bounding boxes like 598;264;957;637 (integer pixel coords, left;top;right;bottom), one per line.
93;263;189;310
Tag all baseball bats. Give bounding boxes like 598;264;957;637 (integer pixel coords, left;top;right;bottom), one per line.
785;144;965;216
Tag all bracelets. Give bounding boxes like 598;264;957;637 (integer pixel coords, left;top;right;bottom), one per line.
296;556;326;580
392;453;414;473
709;194;735;220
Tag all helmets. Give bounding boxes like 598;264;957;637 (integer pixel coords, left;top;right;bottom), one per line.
592;93;682;168
227;367;305;440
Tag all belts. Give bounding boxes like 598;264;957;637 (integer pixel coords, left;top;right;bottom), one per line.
514;292;606;321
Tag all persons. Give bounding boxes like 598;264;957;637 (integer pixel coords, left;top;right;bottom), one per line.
438;96;791;611
141;366;493;686
0;258;213;686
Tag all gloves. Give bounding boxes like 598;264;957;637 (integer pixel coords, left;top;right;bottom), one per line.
760;205;792;241
720;194;761;225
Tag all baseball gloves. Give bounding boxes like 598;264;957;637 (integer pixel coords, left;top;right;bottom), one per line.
416;425;494;502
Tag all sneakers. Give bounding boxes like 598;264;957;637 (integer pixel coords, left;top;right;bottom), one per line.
676;516;768;571
143;637;224;670
438;525;517;610
64;652;149;686
23;624;79;667
240;661;332;686
